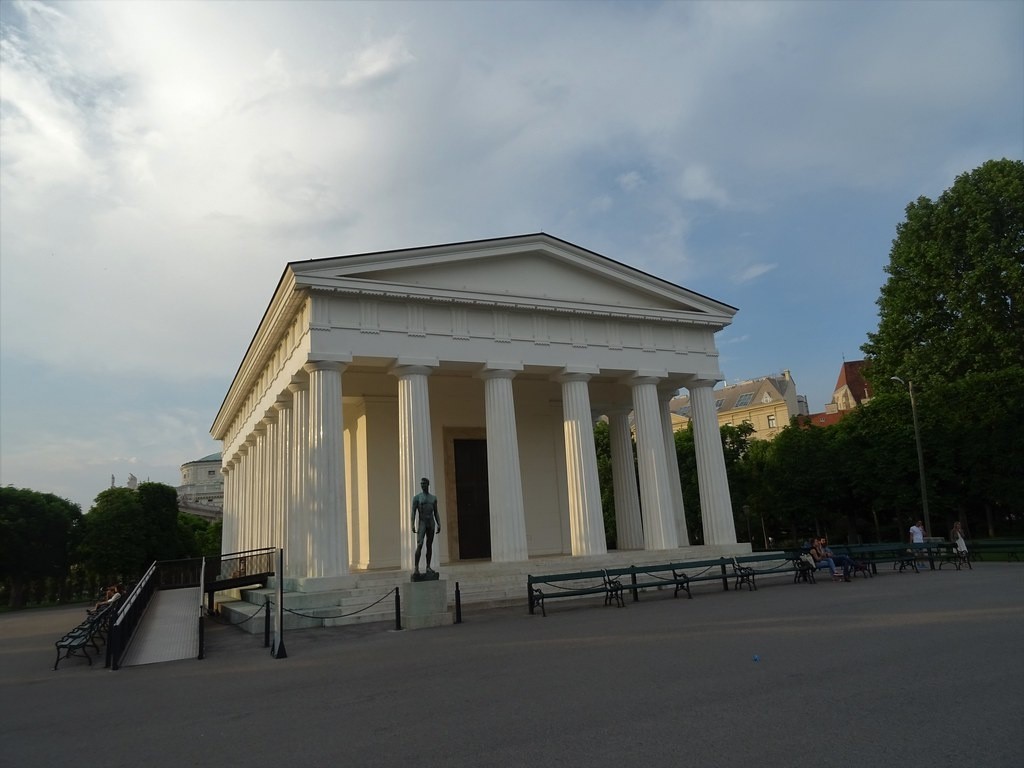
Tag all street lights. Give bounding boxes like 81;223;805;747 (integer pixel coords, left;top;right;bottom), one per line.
890;377;932;538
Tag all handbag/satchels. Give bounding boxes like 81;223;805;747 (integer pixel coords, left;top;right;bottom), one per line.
800;553;815;567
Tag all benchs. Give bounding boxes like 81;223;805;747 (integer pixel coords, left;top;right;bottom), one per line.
527;543;971;616
53;593;123;671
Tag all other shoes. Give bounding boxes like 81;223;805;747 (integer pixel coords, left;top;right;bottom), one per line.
834;572;843;576
845;577;851;581
832;578;841;582
921;564;927;568
917;564;922;568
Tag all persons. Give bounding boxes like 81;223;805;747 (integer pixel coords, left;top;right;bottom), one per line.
909;519;926;568
950;521;969;568
694;520;704;546
809;536;865;582
86;585;126;619
737;514;812;552
410;478;441;576
